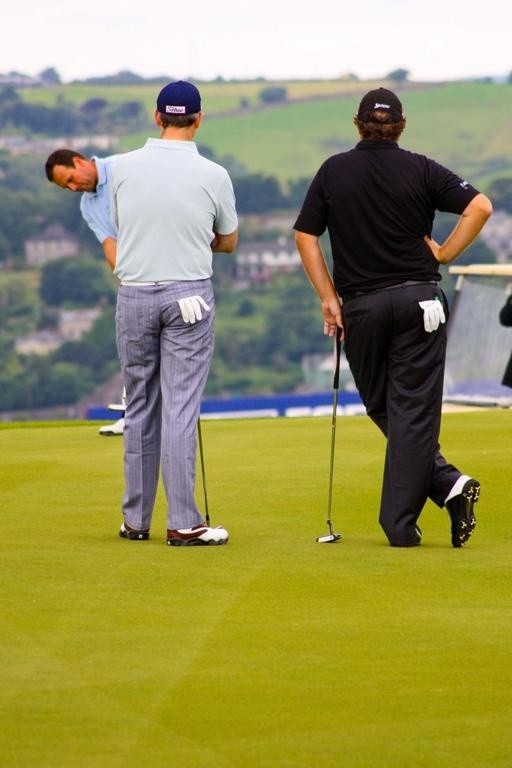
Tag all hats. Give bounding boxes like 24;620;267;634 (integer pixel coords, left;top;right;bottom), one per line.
356;85;407;126
156;79;202;116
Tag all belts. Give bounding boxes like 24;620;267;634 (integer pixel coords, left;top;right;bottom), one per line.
340;278;439;301
119;277;175;288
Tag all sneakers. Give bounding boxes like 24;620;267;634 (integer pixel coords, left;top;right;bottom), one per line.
166;523;230;546
118;519;150;539
445;473;482;548
98;413;125;435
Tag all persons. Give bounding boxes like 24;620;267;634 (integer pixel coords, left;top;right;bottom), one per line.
497;291;512;388
43;147;147;437
292;86;494;549
110;77;242;546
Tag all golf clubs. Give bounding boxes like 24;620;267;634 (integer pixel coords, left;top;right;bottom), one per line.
317;326;342;543
198;416;223;529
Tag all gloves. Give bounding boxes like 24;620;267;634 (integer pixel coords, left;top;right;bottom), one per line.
176;295;212;325
419;297;446;333
